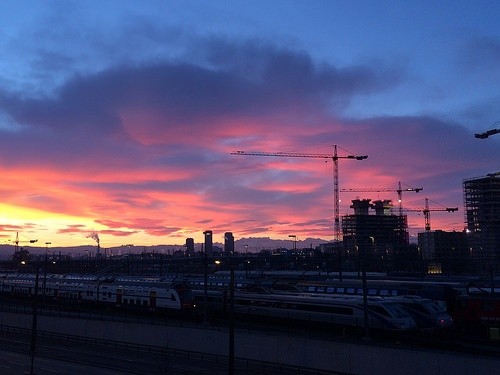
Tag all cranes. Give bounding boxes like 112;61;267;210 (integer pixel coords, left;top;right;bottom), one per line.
385;198;459;231
338;180;425;214
473;121;500;139
230;142;369;242
5;232;39;252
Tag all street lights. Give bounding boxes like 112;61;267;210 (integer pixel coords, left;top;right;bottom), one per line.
243;243;248;255
289;234;297;268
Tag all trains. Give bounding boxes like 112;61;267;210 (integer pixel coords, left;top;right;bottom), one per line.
12;271;499;355
